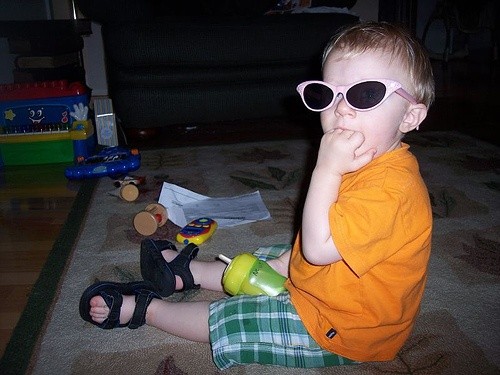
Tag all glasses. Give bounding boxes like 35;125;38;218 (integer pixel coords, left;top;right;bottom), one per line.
296;78;419;131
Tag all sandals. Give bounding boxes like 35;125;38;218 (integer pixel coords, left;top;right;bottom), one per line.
140;238;201;298
79;280;164;330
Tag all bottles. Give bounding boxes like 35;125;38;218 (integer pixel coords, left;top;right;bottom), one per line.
218;253;288;296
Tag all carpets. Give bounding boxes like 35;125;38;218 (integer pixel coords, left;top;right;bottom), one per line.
0;127;500;375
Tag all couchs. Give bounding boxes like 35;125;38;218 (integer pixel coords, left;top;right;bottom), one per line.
106;13;359;129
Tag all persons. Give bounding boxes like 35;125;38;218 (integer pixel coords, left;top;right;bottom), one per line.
78;20;435;370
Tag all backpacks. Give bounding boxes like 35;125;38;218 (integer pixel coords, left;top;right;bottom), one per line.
423;0;497;114
